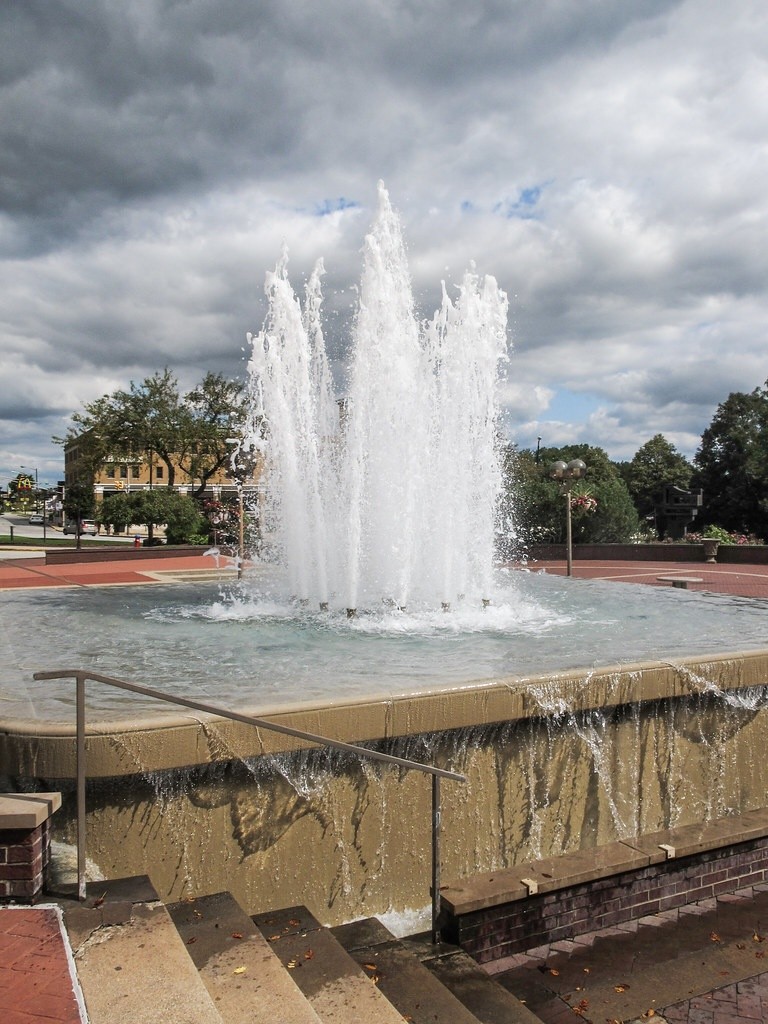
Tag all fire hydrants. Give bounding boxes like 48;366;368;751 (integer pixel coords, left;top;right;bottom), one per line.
134;535;140;547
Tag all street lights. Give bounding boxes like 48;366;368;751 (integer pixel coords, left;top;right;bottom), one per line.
45;482;66;526
549;459;587;577
20;465;41;514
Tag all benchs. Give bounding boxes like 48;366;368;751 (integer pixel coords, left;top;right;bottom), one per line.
656;576;703;589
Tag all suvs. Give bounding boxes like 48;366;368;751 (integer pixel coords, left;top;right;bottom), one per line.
63;520;98;536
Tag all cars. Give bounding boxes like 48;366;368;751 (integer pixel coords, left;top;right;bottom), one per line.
29;514;44;524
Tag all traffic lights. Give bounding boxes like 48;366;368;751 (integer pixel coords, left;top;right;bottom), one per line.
118;481;123;488
114;481;119;491
